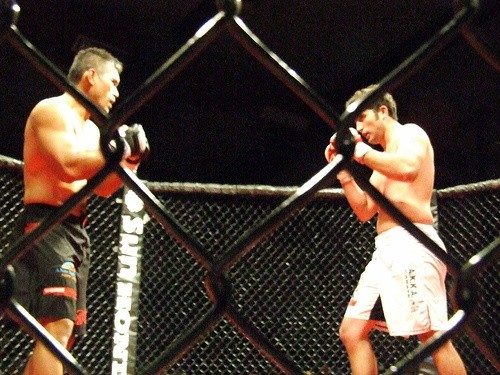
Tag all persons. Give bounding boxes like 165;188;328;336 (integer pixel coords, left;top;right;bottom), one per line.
22;48;148;375
325;85;468;375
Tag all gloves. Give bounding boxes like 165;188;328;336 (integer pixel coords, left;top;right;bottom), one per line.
330;126;373;164
324;143;353;185
108;138;131;162
123;123;150;164
